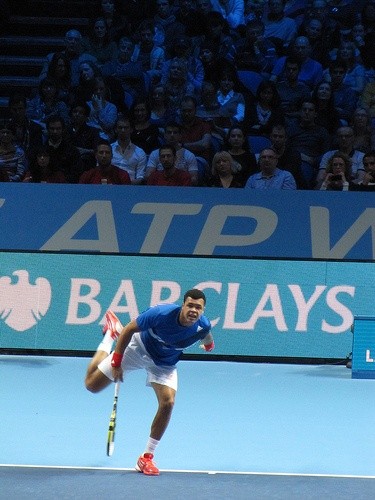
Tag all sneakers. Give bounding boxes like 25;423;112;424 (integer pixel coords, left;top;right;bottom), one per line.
102;310;124;342
136;453;160;475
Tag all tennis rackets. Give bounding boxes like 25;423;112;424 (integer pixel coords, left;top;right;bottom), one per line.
107;378;120;456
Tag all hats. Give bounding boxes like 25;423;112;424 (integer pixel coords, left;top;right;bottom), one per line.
174;35;190;47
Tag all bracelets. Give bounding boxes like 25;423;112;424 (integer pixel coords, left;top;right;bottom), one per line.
204;340;214;352
112;352;123;367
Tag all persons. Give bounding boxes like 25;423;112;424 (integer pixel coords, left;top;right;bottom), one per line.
0;0;243;186
207;0;375;192
86;288;215;476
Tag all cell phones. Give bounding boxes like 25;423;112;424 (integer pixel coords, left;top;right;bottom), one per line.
330;175;342;181
